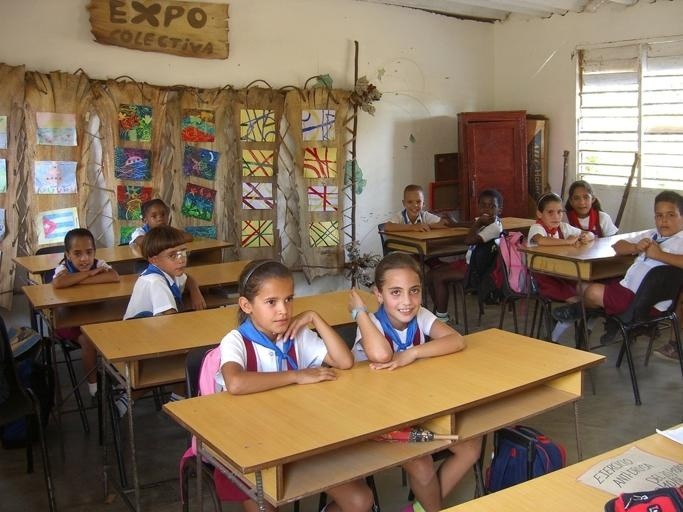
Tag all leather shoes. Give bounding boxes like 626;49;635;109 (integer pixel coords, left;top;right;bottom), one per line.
551;302;582;321
600;319;619;345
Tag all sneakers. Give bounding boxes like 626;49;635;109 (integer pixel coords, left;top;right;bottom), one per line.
653;340;680;362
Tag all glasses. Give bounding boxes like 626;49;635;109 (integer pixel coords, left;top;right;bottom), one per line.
168;250;190;261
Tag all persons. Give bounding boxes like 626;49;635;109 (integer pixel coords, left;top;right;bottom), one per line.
129;197;195;247
111;223;208;423
551;190;683;346
654;290;683;363
52;227;120;399
383;183;452;313
427;188;504;325
211;259;375;512
526;191;596;346
350;249;485;512
561;179;620;237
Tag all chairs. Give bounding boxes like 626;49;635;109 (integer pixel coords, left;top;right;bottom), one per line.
334;324;487;499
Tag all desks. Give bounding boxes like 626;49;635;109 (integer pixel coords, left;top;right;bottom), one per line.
436;422;683;512
163;327;607;512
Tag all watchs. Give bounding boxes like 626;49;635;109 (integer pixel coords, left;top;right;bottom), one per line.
350;305;368;322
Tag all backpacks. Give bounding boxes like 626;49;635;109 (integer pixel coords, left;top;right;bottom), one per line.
190;336;298;463
470;232;536;305
485;426;565;492
